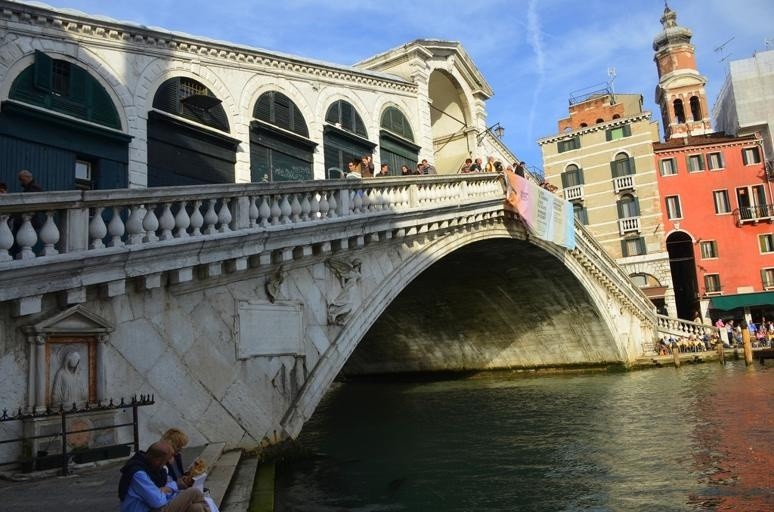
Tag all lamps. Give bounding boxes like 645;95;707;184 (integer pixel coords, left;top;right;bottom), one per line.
475;123;505;148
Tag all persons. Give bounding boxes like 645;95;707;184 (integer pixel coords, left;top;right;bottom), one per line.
118;439;211;512
10;169;42;260
657;303;774;354
159;428;220;512
346;150;558;195
0;181;14;231
